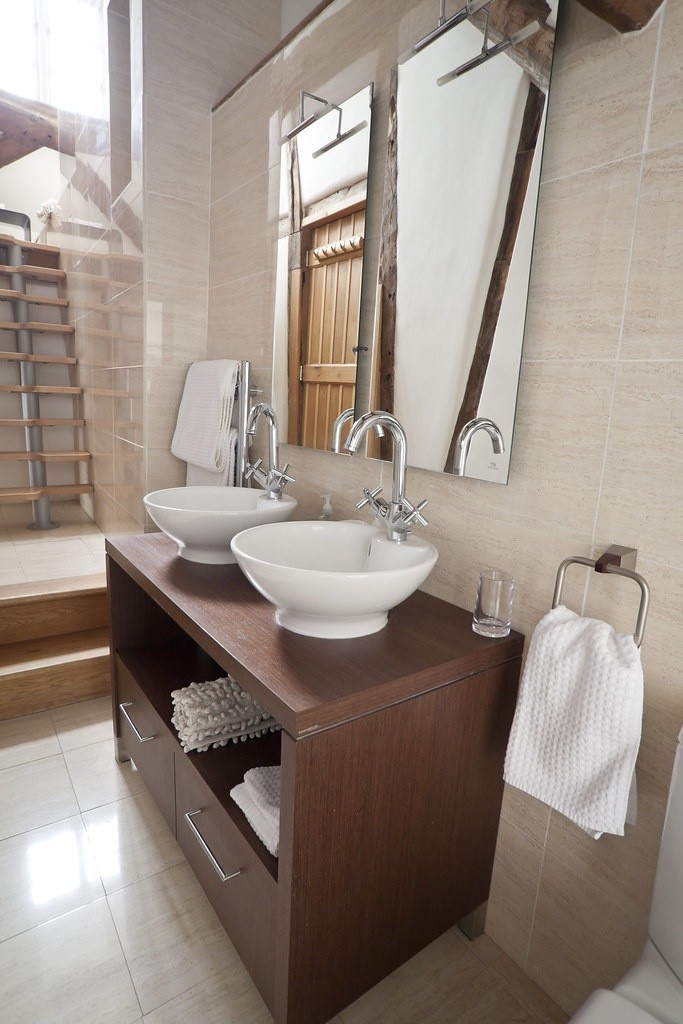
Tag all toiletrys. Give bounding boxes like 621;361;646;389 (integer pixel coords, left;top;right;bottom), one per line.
316;493;335;521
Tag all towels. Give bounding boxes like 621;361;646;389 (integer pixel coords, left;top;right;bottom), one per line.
502;604;640;841
229;763;281;857
169;359;239;486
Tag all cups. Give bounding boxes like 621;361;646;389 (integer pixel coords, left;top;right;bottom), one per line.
474;570;515;637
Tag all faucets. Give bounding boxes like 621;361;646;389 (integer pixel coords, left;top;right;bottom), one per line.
345;408;406;504
331;408;386;453
247;403;279;484
450;416;506;476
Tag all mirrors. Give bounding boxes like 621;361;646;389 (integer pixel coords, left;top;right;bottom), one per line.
365;0;559;486
272;82;375;457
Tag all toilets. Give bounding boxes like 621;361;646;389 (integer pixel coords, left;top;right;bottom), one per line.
552;724;682;1024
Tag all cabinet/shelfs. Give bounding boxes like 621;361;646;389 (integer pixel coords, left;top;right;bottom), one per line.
105;531;525;1024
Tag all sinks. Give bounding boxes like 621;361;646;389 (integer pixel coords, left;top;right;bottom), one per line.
231;520;440;639
143;486;298;565
